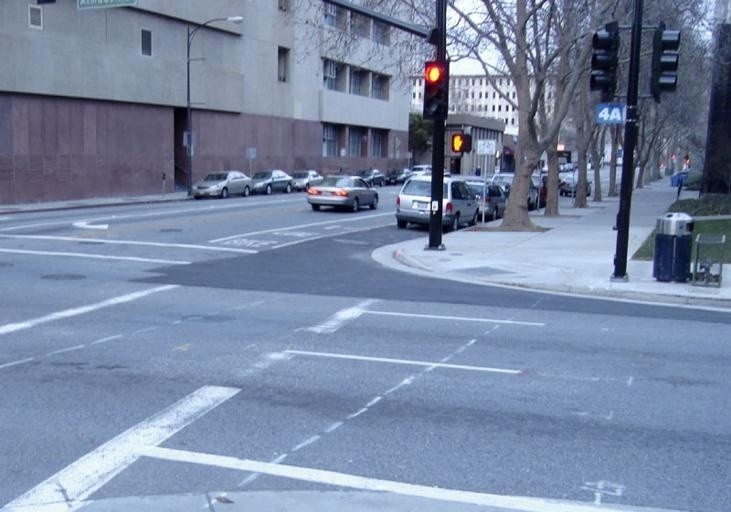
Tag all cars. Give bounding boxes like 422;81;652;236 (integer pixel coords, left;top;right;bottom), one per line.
541;155;598;174
189;164;453;197
561;176;595;199
307;175;380;212
398;174;551;233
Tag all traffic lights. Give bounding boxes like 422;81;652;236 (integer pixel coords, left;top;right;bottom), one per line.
591;30;611;88
425;63;445;117
452;134;470;153
659;30;681;92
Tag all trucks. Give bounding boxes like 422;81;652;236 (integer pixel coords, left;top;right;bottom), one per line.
604;144;623;167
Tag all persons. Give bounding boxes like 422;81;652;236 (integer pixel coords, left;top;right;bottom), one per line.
680;154;691;190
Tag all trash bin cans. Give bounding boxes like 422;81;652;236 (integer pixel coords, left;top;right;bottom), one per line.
653;212;695;282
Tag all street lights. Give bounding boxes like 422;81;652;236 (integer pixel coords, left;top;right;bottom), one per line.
185;15;245;199
76;0;135;11
